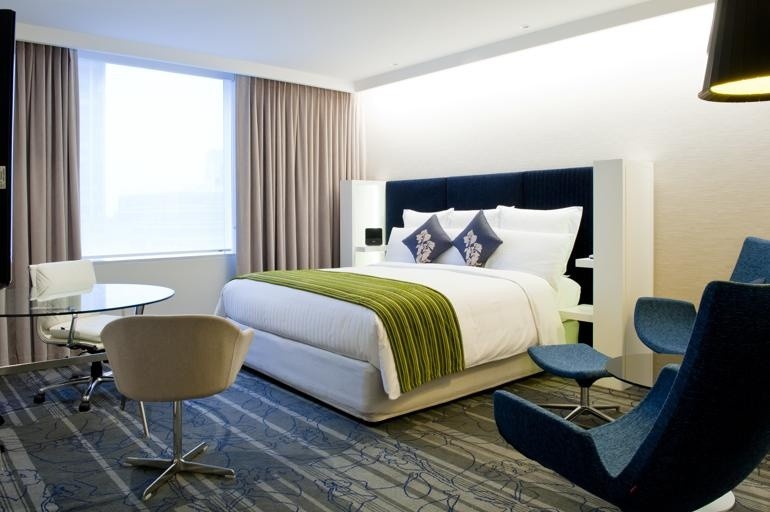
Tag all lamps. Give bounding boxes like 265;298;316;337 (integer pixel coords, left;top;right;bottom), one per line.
700;1;770;106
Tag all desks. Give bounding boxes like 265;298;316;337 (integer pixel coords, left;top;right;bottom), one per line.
0;279;178;377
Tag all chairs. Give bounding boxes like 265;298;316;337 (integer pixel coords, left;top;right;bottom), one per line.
730;237;769;283
492;279;769;511
28;257;151;411
99;315;252;502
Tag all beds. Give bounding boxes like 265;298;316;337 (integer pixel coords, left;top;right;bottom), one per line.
218;166;591;422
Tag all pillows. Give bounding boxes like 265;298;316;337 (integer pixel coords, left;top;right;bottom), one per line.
380;200;584;280
